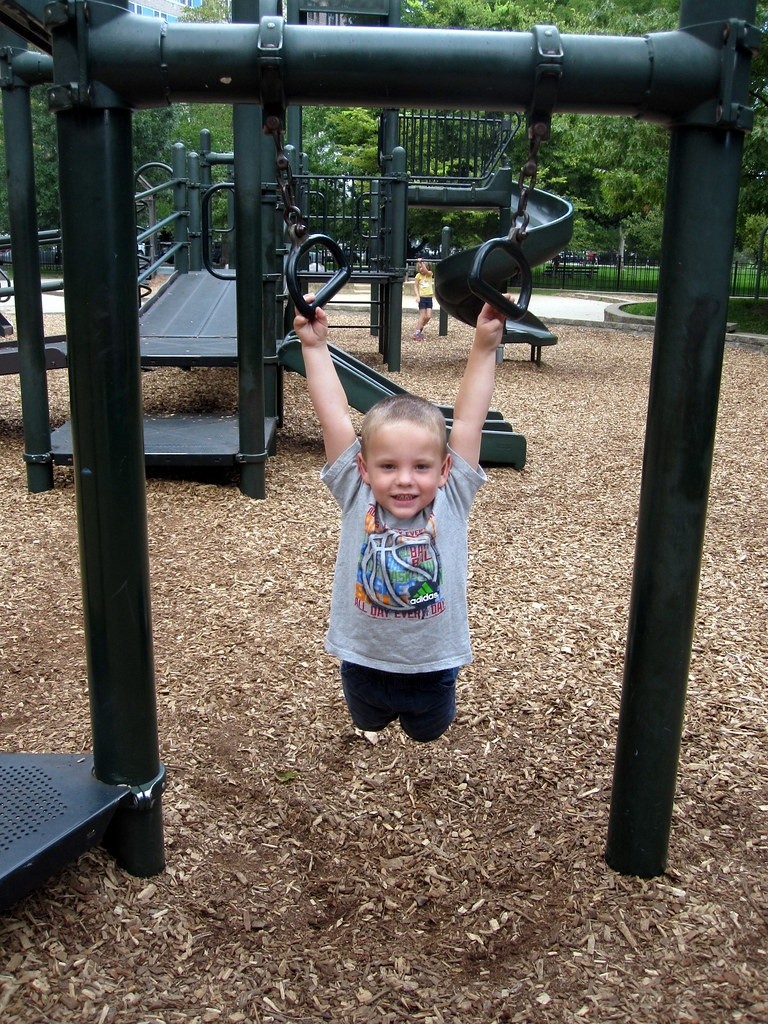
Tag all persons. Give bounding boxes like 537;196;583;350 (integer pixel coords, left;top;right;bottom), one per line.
293;291;515;743
412;258;434;340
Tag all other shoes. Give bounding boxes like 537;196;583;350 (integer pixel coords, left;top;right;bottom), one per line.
412;330;424;340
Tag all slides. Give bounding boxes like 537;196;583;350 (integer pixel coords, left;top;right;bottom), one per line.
433;180;575;347
276;327;528;471
